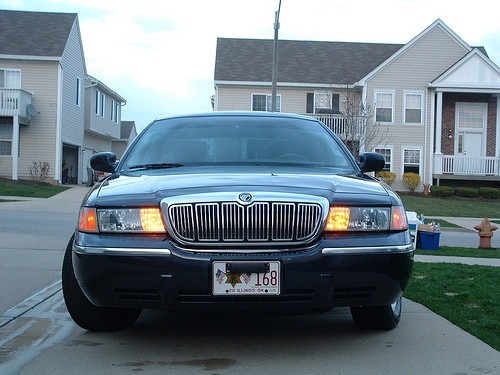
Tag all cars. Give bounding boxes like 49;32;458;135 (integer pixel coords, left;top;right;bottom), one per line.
62;110;415;334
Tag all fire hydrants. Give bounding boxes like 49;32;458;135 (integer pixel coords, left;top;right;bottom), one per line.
474;216;498;249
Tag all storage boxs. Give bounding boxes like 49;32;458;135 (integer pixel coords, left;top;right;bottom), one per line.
417;229;441;250
